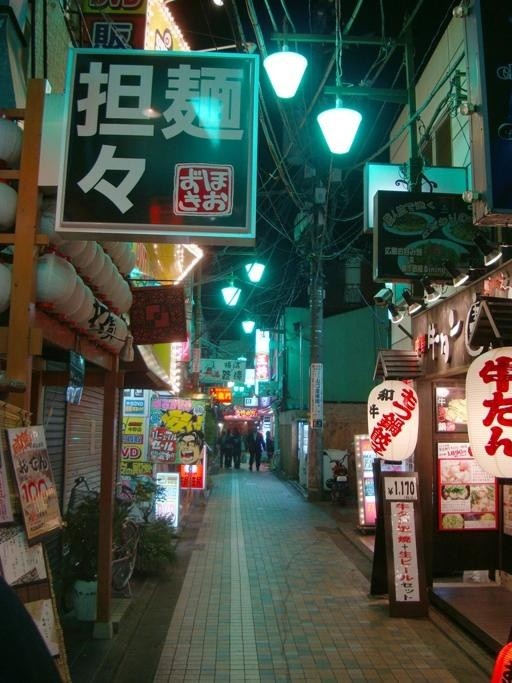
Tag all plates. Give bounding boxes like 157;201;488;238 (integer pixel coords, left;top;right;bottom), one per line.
383;211;439;237
441;220;493;246
398;238;471;276
444;407;468;425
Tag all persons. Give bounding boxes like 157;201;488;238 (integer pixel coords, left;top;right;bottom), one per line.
177;430;204;464
218;425;274;472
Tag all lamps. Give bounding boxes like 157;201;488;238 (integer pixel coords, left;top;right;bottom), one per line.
384;239;503;324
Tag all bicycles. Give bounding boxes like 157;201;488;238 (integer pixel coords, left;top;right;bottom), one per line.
60;474;144;594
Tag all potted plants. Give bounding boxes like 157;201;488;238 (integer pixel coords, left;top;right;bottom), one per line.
60;468;178;621
